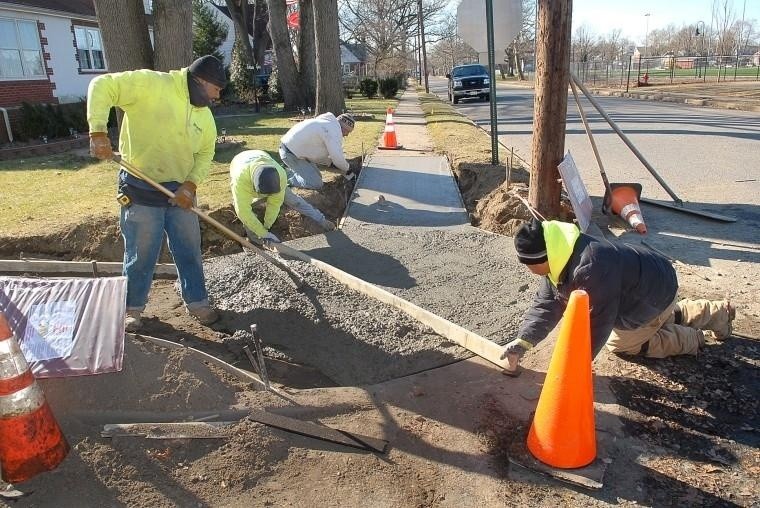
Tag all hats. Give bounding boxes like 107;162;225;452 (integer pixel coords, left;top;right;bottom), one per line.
514;219;549;263
254;166;282;198
190;54;230;87
337;111;356;131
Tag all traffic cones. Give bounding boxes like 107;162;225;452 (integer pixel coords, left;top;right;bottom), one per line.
602;180;648;237
379;107;403;150
508;292;617;495
0;312;93;503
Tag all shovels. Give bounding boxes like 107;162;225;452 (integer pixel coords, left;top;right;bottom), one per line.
570;77;627;230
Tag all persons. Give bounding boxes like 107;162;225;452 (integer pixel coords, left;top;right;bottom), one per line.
229;150;334;249
279;112;357;190
86;55;227;332
500;219;735;373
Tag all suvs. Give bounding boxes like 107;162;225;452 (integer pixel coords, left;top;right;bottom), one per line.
445;64;491;104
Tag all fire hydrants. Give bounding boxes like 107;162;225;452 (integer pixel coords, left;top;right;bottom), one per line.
643;73;650;83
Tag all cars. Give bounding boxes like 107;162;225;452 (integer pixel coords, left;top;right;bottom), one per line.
413;71;422;80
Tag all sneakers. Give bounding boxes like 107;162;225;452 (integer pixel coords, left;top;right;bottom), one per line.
125;304;146;333
716;298;735;343
185;297;220;326
694;328;704;354
320;220;336;231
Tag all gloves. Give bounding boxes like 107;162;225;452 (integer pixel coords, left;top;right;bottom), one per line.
170;181;198;207
266;233;281;245
338;163;358;182
500;341;529;364
89;132;114;161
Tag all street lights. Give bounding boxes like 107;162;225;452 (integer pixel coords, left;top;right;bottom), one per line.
693;19;704;77
644;11;652;73
